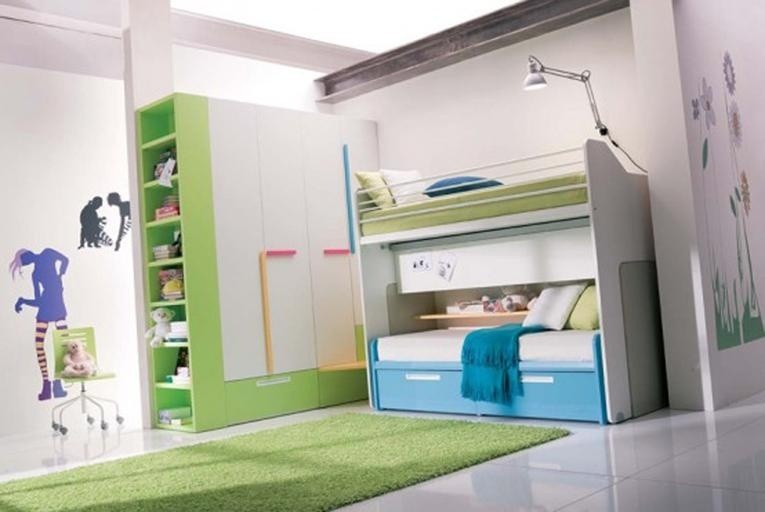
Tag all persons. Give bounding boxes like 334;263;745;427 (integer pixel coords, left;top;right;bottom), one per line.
8;247;70;400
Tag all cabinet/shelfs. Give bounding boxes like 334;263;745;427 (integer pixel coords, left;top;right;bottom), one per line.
320;113;380;410
209;97;317;426
135;93;226;434
377;370;599;421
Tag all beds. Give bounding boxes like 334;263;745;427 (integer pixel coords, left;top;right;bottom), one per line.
353;137;666;429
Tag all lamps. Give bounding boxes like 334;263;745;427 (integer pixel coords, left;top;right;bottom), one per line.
523;55;651;175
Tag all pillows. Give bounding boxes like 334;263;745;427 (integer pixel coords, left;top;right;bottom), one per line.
519;280;600;333
354;167;503;210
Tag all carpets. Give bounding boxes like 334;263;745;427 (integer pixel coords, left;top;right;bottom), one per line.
0;408;574;511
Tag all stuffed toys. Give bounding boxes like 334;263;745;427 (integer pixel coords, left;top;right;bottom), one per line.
61;337;104;377
481;283;538;311
144;306;176;348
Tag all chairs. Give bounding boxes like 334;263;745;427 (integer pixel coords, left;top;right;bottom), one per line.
50;325;125;436
